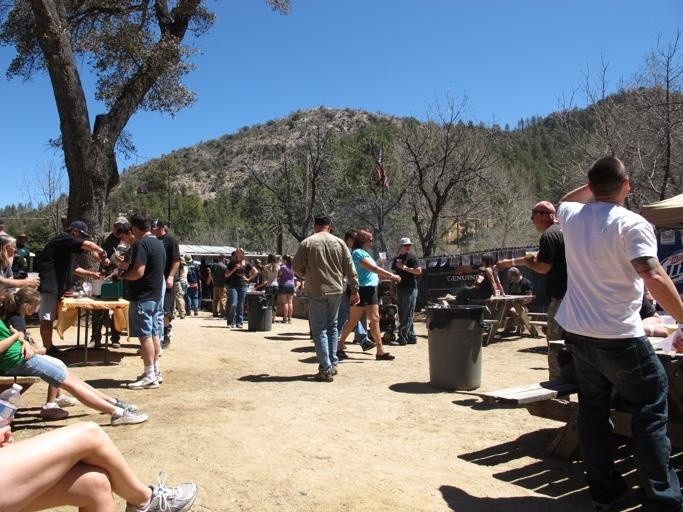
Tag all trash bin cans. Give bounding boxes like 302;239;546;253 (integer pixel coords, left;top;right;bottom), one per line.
424;305;487;390
246;291;273;332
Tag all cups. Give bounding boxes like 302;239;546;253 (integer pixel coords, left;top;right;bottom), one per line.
27;272;40;290
378;252;386;263
495;289;500;296
395;258;403;268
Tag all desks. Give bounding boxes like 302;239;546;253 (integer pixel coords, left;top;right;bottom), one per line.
61;297;130;363
471;294;539;343
546;339;683;464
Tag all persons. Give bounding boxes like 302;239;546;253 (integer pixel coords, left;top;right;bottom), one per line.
0;416;198;511
505;267;534;332
494;200;567;401
0;217;129;357
553;155;683;511
643;325;669;338
150;220;181;348
450;253;505;307
290;211;361;382
1;286;78;420
0;319;148;426
172;250;301;329
114;210;163;389
337;229;421;360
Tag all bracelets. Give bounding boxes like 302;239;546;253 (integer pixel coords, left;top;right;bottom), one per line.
511;257;516;267
672;319;682;332
649;326;655;337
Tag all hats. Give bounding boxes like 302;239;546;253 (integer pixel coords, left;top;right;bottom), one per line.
66;220;91;238
113;216;128;225
182;254;192;262
149;219;165;230
398;237;413;245
506;266;521;281
314;213;332;226
180;257;186;265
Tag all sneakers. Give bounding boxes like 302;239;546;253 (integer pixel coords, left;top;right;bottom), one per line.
44;346;66;359
125;378;159;390
172;310;199;320
407;339;417;344
336;350;349;360
124;480;198;512
54;394;80;406
589;480;638;509
230;323;243;329
280;319;292;324
374;352;395;361
134;372;162;382
360;338;377;351
136;324;172;360
40;402;69;419
389;338;407;346
109;396;149;427
314;367;338;382
85;339;120;349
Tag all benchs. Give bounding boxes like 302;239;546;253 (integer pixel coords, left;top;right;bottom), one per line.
0;375;41;394
482;312;547;345
479;379;577;406
